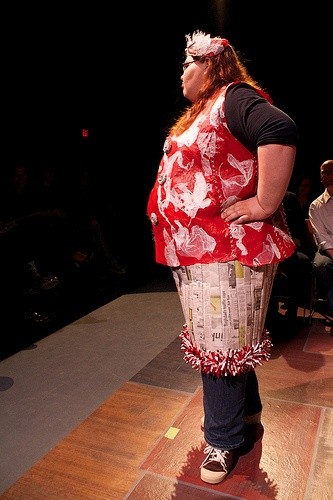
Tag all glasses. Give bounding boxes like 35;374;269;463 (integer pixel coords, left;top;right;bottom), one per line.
181;60;197;69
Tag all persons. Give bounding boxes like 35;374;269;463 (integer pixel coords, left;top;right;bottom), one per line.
145;28;298;484
309;159;333;317
293;175;312;219
267;192;305;330
1;162;130;298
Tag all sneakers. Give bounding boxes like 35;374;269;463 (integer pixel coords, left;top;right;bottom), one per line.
199;443;238;484
199;409;262;429
